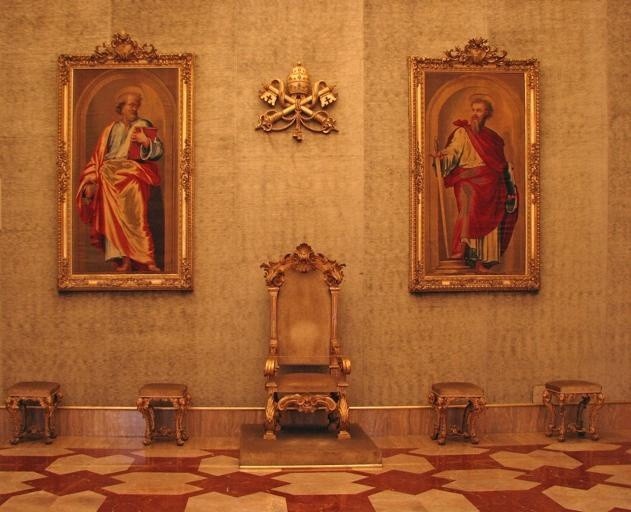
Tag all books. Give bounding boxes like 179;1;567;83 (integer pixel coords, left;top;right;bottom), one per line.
126;125;157;162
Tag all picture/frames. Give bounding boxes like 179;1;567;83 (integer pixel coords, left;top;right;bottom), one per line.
58;32;195;292
406;37;541;292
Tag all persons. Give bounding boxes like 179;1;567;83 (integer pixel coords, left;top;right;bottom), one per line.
430;93;520;273
75;86;163;273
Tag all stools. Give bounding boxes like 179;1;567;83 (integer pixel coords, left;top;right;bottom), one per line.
136;383;190;446
428;382;487;444
543;381;604;442
5;381;60;444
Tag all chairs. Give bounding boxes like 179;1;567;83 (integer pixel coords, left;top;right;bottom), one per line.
258;242;352;441
